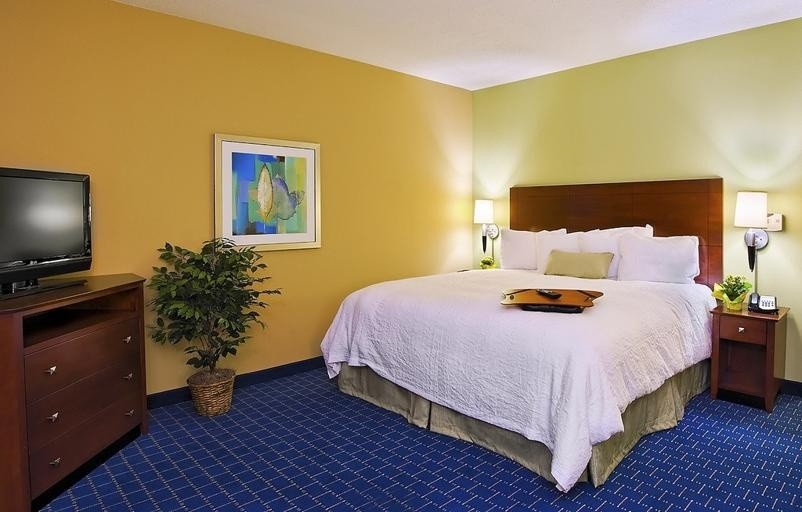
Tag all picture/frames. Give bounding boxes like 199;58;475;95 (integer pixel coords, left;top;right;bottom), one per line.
214;133;322;251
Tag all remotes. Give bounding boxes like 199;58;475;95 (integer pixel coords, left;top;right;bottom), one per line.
535;287;562;299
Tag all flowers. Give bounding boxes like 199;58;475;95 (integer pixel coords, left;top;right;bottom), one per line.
712;274;753;302
479;255;498;270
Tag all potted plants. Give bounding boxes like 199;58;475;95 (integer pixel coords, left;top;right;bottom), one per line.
142;234;284;416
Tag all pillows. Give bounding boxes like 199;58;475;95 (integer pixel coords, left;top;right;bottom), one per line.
534;229;600;274
542;249;614;279
616;235;700;284
500;225;567;270
578;224;653;280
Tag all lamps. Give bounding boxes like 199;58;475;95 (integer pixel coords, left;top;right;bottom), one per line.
473;200;499;253
733;191;770;272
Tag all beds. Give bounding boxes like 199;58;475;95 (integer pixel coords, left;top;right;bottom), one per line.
318;177;724;492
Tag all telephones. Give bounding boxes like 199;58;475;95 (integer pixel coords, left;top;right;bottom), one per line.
748;293;779;315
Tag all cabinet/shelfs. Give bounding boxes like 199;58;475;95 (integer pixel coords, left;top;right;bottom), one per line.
2;273;150;512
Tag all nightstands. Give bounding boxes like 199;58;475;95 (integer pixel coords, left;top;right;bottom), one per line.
710;302;791;414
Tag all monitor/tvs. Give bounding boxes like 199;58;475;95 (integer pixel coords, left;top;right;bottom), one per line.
0;166;92;300
